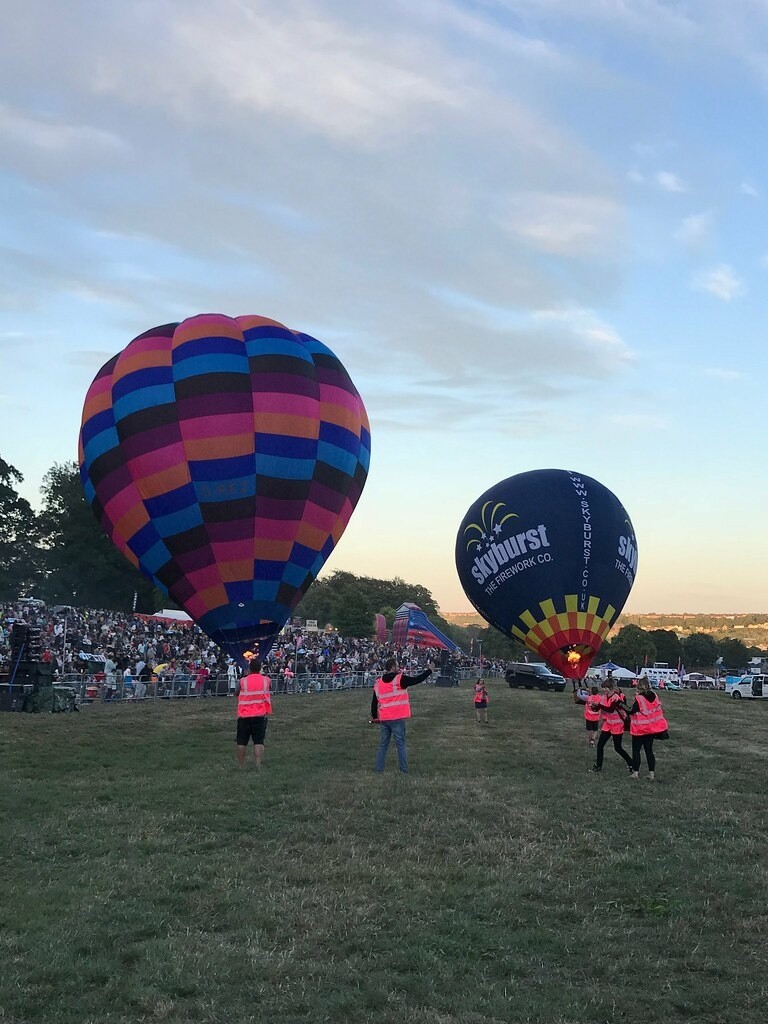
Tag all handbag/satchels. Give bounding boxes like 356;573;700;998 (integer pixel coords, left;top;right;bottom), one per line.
624;718;631;731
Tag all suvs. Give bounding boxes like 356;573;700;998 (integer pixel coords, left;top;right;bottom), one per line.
505;661;566;692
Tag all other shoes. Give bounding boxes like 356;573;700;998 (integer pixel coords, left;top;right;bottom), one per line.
627;766;634;772
629;773;638;779
589;763;601;773
589;740;596;748
646;775;655;780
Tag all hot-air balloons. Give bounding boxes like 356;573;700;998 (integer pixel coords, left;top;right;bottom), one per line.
76;313;371;679
452;468;638;706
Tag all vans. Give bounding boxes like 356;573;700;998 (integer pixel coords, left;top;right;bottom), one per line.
730;673;768;699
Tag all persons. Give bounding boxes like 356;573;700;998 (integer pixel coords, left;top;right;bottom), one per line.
0;601;520;704
473;679;489;723
371;657;435;773
233;661;272;770
584;677;665;783
